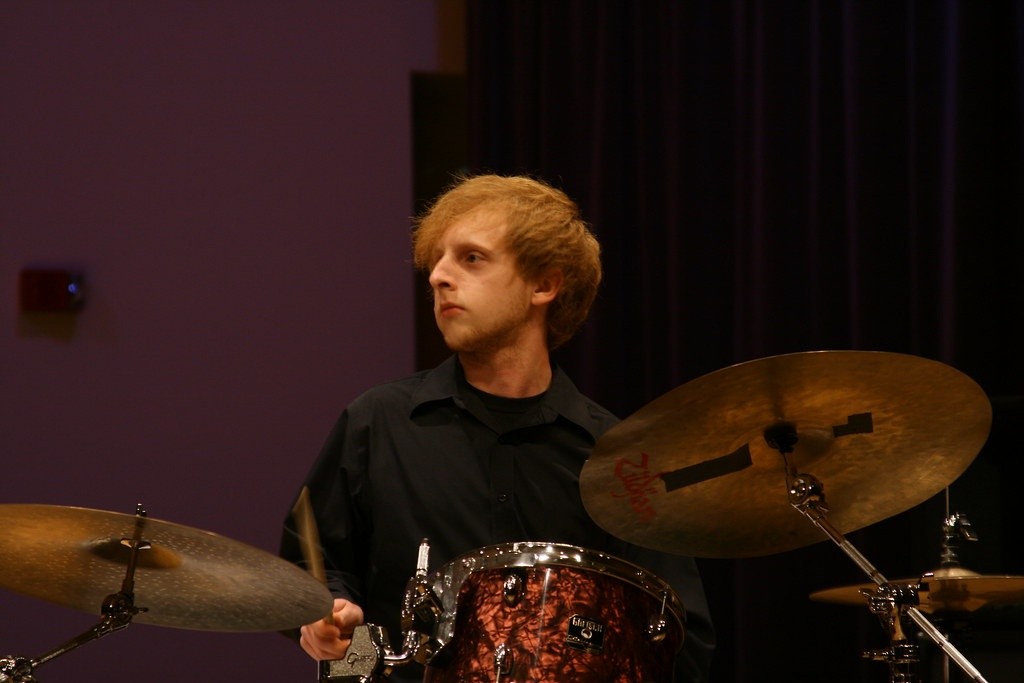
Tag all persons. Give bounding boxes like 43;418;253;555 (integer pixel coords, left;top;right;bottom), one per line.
278;175;728;683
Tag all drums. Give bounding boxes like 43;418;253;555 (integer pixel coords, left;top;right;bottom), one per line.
425;541;688;682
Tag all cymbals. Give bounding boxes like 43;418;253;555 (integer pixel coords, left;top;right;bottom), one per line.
579;347;994;560
1;501;335;635
806;568;1024;617
